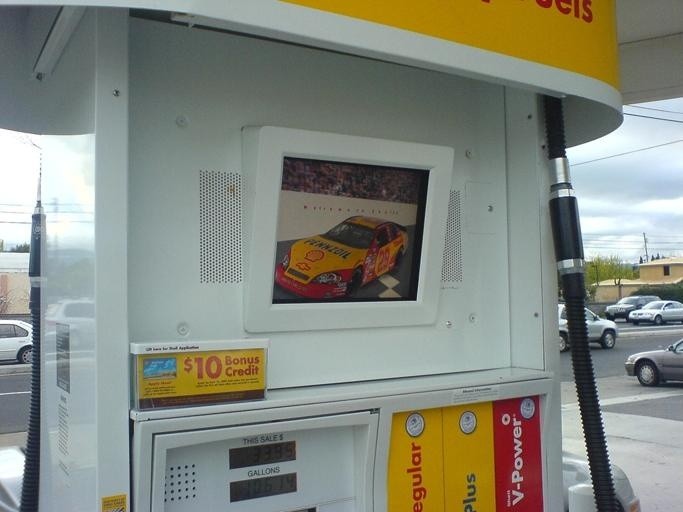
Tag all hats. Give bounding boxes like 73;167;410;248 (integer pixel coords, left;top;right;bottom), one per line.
277;215;407;299
0;446;26;512
558;293;682;390
0;317;32;364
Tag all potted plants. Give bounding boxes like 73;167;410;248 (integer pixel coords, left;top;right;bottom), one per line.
245;125;454;333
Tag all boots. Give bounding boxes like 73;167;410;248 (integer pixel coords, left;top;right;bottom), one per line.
45;299;94;333
